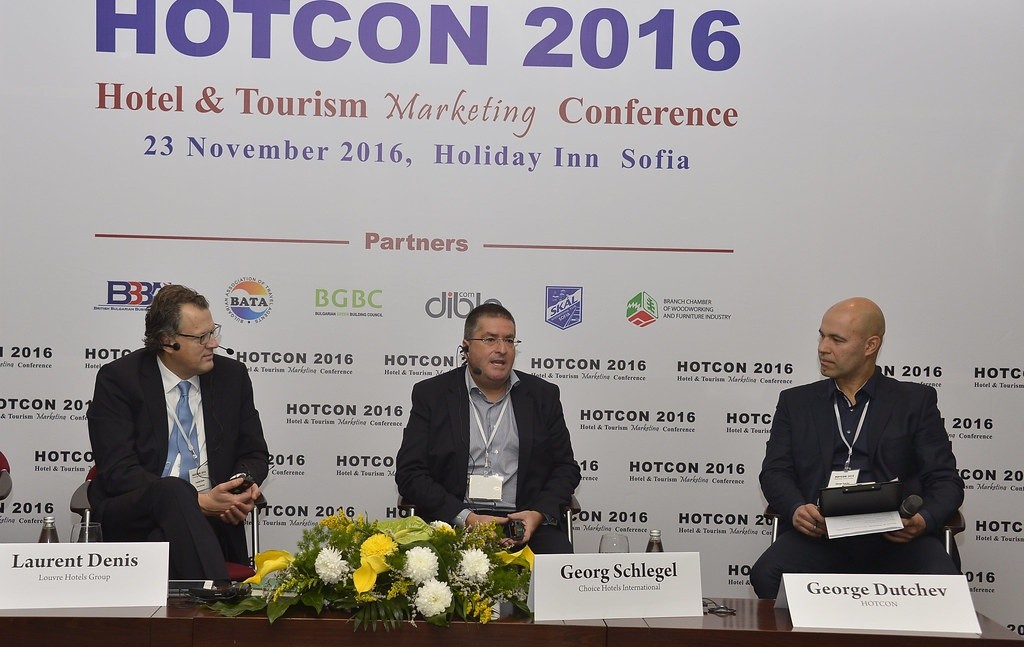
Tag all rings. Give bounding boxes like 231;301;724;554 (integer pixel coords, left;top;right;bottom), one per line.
813;525;818;533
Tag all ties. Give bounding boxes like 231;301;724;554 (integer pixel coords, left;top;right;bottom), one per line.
162;380;200;484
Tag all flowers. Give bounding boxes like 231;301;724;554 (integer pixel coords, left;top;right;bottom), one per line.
198;507;535;633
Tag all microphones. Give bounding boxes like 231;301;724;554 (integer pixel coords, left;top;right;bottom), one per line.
462;347;482;375
219;346;234;355
900;495;923;519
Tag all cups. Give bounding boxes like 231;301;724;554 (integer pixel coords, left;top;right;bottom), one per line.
599;534;630;553
70;522;103;542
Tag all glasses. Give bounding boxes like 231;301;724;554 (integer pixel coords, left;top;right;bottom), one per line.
466;337;522;348
177;323;221;344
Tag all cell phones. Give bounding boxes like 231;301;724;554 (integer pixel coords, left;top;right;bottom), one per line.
168;579;213;594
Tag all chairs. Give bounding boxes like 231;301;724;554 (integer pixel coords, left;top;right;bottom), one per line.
762;504;965;556
0;451;12;501
397;495;581;546
70;465;268;581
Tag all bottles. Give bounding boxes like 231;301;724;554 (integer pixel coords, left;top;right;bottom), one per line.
645;529;665;553
37;516;59;543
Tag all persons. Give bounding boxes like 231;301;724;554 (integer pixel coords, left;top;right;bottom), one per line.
749;296;966;600
86;285;269;582
395;303;582;554
